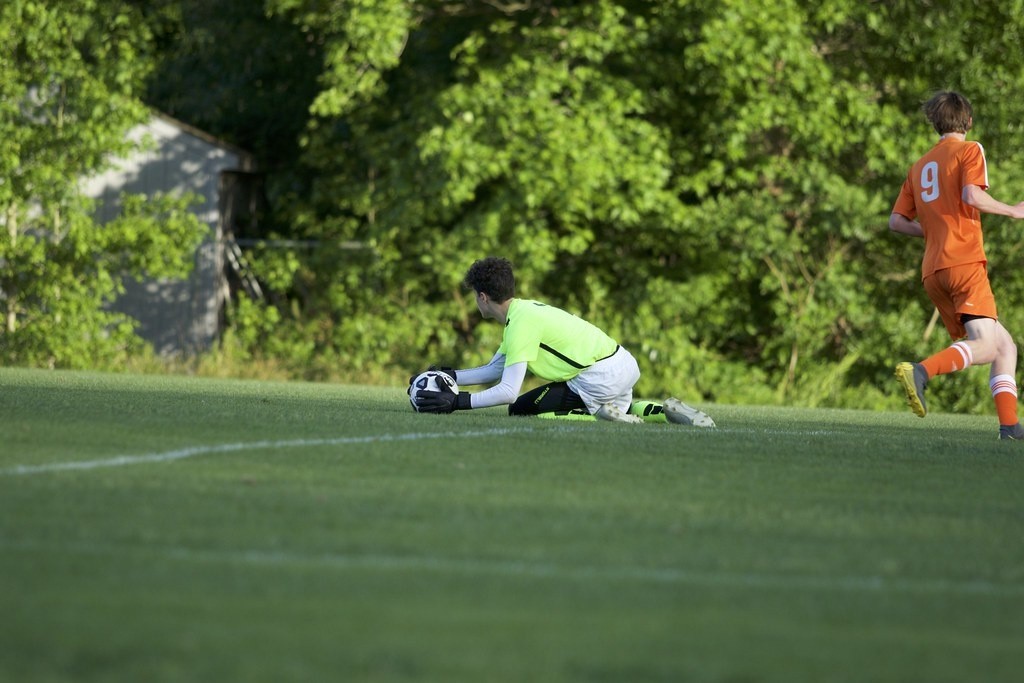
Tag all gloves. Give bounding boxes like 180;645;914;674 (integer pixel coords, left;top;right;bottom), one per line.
415;376;473;414
407;365;457;395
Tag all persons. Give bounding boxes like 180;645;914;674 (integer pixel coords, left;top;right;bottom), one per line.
890;92;1024;439
407;258;715;427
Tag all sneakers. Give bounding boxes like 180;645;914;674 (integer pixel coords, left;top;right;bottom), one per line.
596;402;645;423
894;362;928;418
998;421;1024;440
663;397;716;428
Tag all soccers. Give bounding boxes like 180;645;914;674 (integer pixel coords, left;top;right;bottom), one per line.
409;370;462;414
